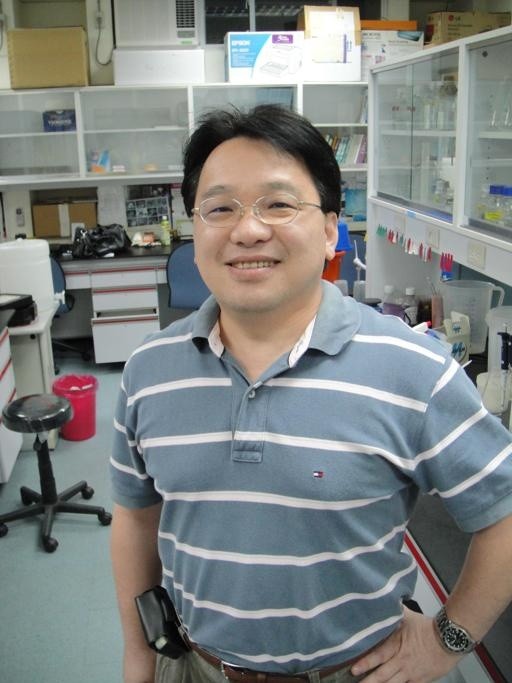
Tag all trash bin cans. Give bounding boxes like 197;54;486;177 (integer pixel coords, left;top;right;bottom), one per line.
52;373;99;442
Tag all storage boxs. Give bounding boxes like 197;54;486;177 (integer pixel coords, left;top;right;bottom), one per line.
6;24;90;89
33;201;97;238
424;11;511;47
361;28;424;69
298;5;363;82
223;30;306;83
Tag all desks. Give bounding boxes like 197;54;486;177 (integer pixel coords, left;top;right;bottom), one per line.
48;238;192;367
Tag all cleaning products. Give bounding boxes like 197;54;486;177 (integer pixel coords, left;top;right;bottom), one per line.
481;331;512;434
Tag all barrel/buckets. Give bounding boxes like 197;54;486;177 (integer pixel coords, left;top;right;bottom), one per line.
321;250;347;284
0;238;54;312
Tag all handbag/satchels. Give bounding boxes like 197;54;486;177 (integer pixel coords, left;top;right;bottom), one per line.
71;222;132;260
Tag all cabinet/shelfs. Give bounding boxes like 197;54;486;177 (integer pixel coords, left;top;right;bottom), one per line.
463;23;511;250
297;81;365;232
0;85;86;185
366;41;463;230
6;300;62;453
1;325;25;485
190;82;301;140
79;84;195;181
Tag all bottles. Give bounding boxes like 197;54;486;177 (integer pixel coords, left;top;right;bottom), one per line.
381;283;419;326
159;214;171;246
433;177;454;208
485;183;512;228
488;70;512;131
390;85;456;129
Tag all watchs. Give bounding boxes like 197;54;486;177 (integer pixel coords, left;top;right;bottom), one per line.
432;606;480;654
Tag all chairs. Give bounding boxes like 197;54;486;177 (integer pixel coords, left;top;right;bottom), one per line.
49;254;91;374
166;240;212;309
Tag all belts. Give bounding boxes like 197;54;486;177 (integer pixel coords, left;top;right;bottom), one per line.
176;623;407;683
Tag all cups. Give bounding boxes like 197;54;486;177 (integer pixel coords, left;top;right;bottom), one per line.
484;306;511;375
440;279;507;356
476;370;510;418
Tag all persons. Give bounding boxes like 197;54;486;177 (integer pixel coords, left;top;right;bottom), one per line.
106;99;510;682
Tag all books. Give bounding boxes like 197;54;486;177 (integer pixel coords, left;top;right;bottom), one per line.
324;130;370;167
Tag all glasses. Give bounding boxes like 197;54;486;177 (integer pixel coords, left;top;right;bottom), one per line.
191;190;330;230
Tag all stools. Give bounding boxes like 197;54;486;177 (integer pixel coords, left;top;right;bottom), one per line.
1;393;112;553
